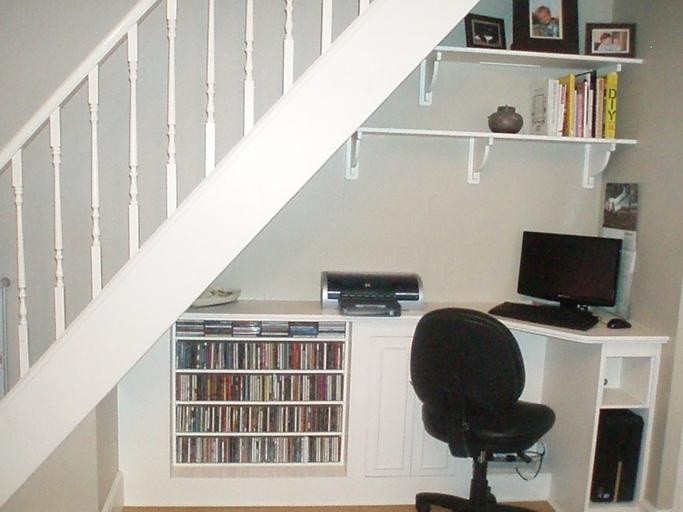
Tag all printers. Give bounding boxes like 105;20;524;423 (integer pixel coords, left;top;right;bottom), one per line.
320;270;424;316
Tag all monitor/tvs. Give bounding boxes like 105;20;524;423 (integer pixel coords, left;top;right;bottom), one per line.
518;231;622;316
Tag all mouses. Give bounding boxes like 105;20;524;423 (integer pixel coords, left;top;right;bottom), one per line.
607;319;631;328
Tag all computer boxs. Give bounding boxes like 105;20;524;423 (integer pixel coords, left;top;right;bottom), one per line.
590;409;644;501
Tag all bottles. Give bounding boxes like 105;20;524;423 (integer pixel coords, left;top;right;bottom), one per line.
489;105;525;133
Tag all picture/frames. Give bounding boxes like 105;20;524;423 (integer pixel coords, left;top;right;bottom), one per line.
508;0;580;57
581;20;639;59
463;11;506;49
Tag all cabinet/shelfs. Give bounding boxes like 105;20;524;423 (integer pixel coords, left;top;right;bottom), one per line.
343;43;644;189
115;297;354;507
344;304;476;507
484;300;671;511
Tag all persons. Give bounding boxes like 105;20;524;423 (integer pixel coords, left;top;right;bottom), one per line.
597;33;622;52
531;6;560;37
604;184;636;215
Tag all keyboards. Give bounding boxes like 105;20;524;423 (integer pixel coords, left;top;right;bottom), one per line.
488;301;599;331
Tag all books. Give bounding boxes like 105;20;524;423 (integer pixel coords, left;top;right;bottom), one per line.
176;322;345;464
531;70;618;138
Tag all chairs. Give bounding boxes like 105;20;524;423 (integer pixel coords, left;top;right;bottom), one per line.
406;305;559;511
